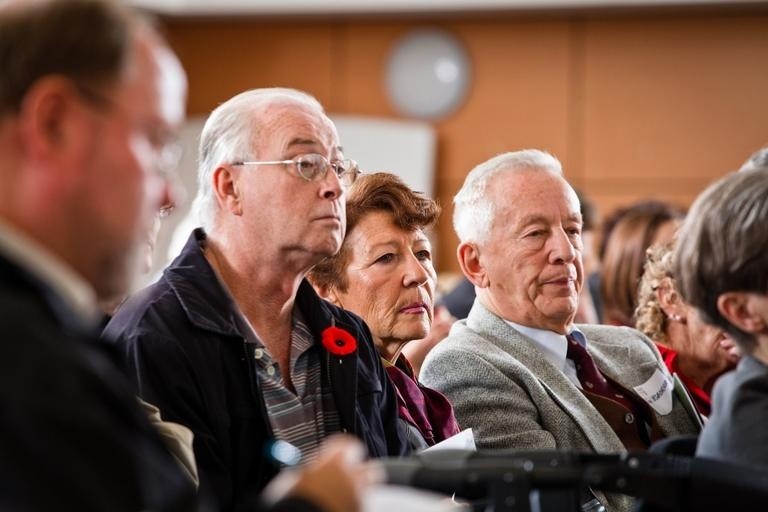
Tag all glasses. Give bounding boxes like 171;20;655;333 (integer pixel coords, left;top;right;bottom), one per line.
232;153;362;187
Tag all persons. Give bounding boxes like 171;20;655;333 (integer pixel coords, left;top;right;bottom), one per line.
1;2;389;512
94;81;477;511
411;142;703;512
628;224;743;428
669;141;767;511
592;199;690;333
399;305;463;376
431;185;598;330
302;164;476;458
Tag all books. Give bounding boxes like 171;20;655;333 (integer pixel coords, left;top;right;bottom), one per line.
415;424;479;466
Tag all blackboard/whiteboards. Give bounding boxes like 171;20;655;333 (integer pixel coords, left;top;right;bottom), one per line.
142;114;436;314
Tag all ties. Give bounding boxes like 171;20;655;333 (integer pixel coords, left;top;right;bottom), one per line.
566;334;656;458
